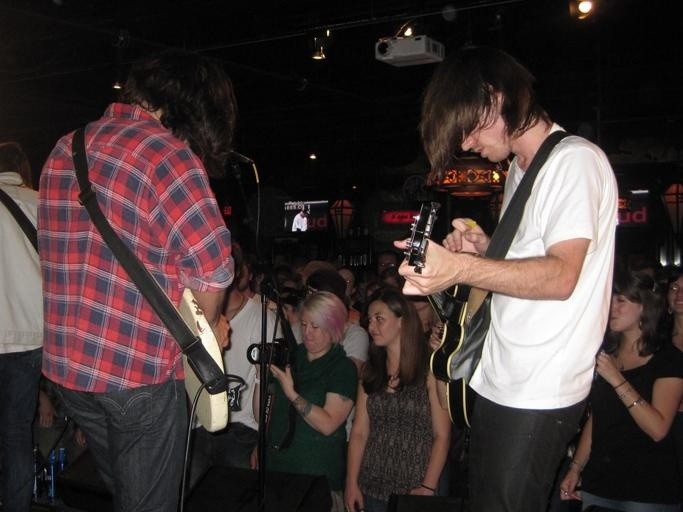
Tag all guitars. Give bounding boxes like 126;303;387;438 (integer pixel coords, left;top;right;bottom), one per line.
175;288;228;433
404;200;495;429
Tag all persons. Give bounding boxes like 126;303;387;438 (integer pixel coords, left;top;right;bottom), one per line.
291;204;312;234
33;381;90;479
35;34;241;510
180;238;683;512
1;141;44;511
395;48;619;510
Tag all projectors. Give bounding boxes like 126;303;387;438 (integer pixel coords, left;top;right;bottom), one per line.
375;35;445;67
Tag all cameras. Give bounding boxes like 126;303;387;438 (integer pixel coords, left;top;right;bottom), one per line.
247;338;294;366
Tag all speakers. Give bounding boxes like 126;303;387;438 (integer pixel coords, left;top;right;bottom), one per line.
178;464;332;512
